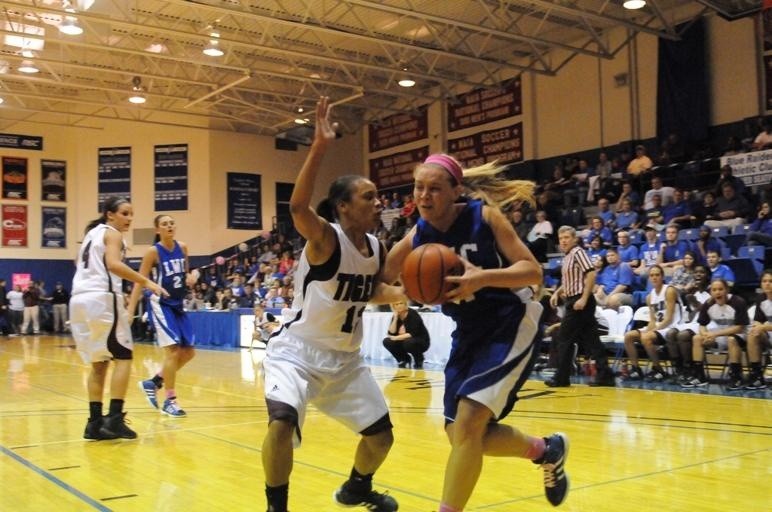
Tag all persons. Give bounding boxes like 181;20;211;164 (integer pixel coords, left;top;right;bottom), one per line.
257;93;410;512
68;195;171;440
184;129;772;389
125;213;196;417
379;152;572;512
0;279;68;336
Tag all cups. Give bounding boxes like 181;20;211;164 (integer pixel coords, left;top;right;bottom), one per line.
206;303;211;308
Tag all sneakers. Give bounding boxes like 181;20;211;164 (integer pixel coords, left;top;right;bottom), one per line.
724;374;768;391
137;378;161;412
161;396;187;416
333;477;400;512
534;431;571;508
668;372;712;389
629;367;666;381
588;376;616;387
397;355;426;370
100;410;141;440
544;376;572;388
83;414;105;440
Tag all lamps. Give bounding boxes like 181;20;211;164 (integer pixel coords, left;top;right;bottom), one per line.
203;37;225;57
59;18;85;36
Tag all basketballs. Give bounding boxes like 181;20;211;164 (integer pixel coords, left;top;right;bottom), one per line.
402;242;462;304
